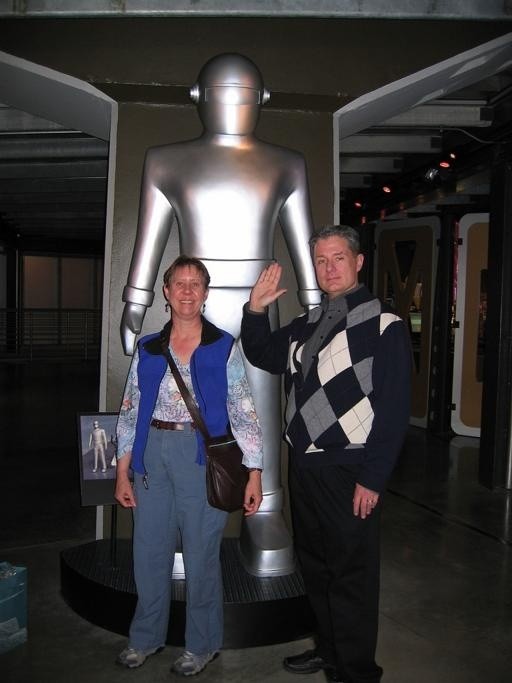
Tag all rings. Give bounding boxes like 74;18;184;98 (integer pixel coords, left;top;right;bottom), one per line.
366;499;374;503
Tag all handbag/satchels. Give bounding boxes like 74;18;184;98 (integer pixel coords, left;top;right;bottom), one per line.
203;433;249;514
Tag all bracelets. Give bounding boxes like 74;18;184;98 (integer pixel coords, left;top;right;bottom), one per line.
246;467;263;473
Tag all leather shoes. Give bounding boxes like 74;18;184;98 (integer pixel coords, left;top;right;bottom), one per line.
283;648;323;673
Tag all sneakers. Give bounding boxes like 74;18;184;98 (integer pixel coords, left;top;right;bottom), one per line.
117;641;166;670
172;647;220;676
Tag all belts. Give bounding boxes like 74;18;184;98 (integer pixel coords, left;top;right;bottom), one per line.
151;417;196;431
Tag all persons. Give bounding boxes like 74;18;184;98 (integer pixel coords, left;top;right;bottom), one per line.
241;223;412;682
114;255;264;678
